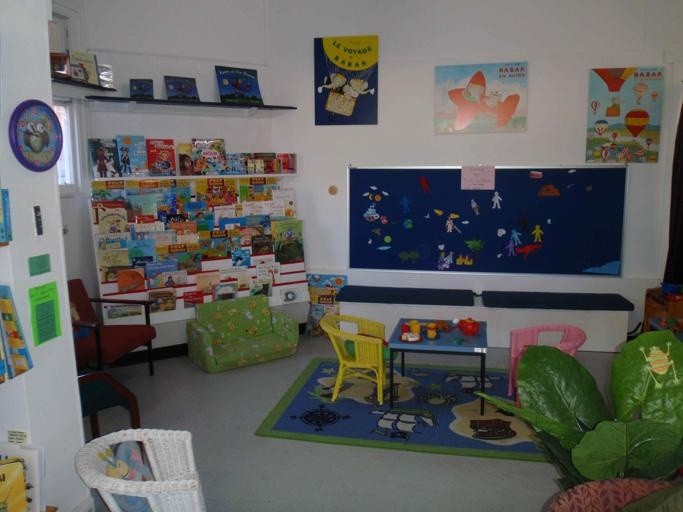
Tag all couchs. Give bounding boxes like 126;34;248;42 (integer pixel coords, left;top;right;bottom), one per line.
186;294;300;374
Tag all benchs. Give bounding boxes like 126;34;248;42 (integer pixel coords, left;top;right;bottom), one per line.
335;285;635;352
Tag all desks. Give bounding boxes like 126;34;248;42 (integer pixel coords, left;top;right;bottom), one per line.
387;318;487;416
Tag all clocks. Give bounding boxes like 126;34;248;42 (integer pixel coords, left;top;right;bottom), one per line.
9;99;63;172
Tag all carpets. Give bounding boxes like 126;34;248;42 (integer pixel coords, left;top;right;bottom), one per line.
253;358;547;462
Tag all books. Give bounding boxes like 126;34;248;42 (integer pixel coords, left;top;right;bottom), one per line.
88;136;306;318
164;75;200;102
214;65;264;106
67;49;101;87
129;78;153;98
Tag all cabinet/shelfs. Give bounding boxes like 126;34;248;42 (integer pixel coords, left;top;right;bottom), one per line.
88;173;312;370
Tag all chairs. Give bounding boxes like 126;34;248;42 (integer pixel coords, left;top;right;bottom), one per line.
319;311;384;404
67;278;208;512
508;323;587;409
